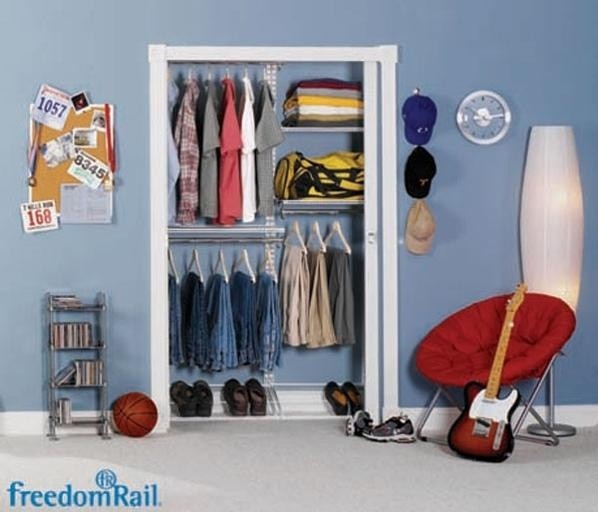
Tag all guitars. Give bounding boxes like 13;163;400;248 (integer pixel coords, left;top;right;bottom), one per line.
446;284;529;462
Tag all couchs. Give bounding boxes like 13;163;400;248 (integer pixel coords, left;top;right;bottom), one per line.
415;293;576;449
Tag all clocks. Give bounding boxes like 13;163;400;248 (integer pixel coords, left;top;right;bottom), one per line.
456;92;512;146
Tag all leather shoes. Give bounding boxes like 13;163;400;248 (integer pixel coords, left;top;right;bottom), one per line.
171;378;266;416
325;381;348;415
342;382;363;413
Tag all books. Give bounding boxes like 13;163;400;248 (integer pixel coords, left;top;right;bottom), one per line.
47;294;103;423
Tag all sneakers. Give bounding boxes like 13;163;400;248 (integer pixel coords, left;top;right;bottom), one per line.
345;410;373;437
361;415;416;443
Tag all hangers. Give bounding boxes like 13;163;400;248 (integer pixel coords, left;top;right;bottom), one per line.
169;63;277;88
289;219;357;255
166;239;281;284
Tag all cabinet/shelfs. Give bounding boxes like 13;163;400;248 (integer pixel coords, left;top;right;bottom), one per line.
275;125;365;223
43;290;110;440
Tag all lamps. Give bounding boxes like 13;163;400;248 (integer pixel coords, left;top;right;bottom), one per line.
521;125;586;437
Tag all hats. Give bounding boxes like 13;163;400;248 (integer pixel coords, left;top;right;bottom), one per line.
404;147;436;199
400;95;437;144
405;199;434;255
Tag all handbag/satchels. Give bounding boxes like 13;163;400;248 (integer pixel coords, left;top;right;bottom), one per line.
274;151;364;200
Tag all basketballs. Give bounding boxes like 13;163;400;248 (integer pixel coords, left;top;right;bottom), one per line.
110;393;157;438
